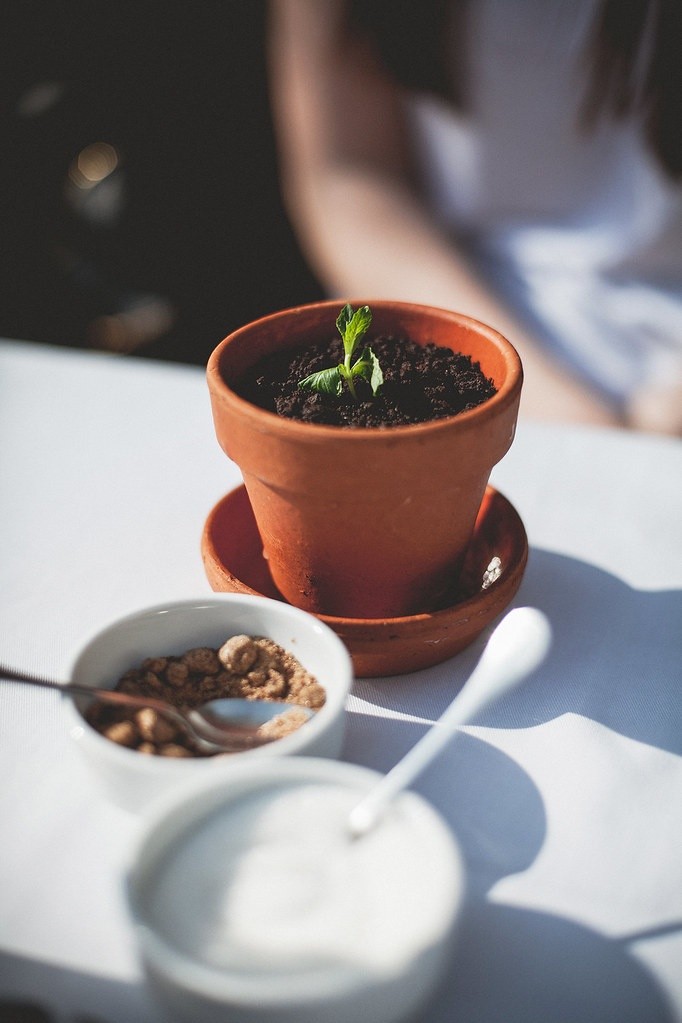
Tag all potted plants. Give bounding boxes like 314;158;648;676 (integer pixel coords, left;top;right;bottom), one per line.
200;298;530;676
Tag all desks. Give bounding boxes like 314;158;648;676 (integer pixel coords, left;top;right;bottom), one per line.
0;339;682;1023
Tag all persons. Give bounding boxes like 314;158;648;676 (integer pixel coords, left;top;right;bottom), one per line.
262;0;682;438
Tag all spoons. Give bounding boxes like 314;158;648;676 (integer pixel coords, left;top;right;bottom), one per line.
0;662;318;754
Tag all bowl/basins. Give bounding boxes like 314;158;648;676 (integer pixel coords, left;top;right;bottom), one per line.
110;753;468;1023
57;591;353;815
